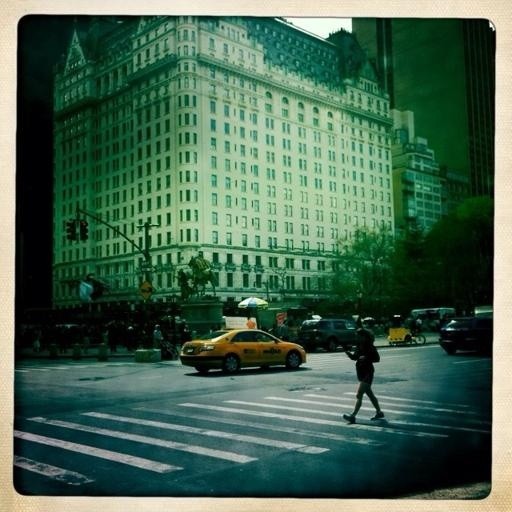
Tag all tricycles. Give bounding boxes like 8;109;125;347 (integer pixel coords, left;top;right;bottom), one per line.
387;315;426;347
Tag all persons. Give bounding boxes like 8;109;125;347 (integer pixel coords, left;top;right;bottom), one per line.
153;324;163;349
179;322;191;344
342;328;384;424
27;319;120;354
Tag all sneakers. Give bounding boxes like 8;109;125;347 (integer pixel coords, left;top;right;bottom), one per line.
342;411;385;424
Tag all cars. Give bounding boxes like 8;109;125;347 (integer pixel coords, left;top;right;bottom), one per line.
438;315;493;355
179;319;307;376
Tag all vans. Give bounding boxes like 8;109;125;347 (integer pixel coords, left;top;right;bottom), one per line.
298;317;361;352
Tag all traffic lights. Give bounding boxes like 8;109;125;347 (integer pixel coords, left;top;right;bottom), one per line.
65;219;89;241
